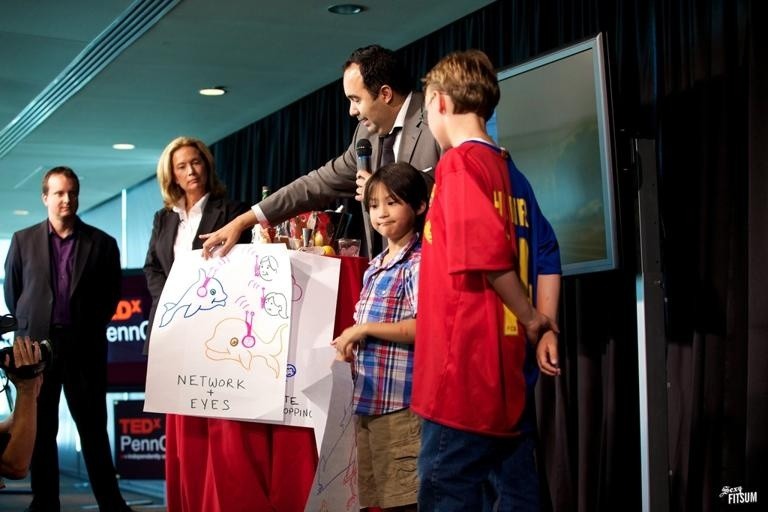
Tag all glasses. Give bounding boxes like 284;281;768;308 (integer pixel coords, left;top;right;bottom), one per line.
417;94;434;124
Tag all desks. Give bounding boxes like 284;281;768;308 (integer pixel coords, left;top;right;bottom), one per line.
163;255;369;512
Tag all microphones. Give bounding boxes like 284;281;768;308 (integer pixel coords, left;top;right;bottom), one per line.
356;137;375;220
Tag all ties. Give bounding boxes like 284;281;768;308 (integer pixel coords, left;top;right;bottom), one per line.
379;127;400;167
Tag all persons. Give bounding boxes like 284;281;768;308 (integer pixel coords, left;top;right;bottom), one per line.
141;137;253;357
3;166;136;512
1;335;48;481
197;43;447;262
407;50;566;512
330;158;428;512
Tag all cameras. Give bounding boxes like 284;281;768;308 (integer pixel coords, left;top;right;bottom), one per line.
0;338;54;369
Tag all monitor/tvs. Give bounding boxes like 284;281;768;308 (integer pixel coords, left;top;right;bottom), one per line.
482;29;619;277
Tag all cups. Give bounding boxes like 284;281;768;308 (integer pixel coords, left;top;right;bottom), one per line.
338;239;361;257
